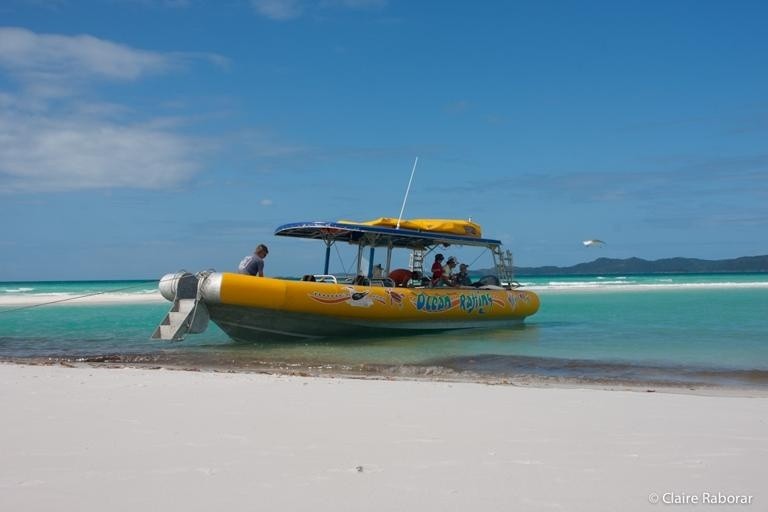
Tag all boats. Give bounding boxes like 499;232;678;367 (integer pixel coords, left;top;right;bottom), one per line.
148;217;540;343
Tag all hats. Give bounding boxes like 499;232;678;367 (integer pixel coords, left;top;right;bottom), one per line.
435;254;444;261
460;264;469;270
446;256;460;264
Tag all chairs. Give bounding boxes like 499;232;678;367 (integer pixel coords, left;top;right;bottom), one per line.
479;275;499;287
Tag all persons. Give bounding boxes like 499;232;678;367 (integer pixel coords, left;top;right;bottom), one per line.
457;263;470;286
431;253;446;285
387;268;423;289
237;243;269;278
443;256;457;277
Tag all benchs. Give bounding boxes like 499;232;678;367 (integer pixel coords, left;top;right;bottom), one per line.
314;275;395;287
